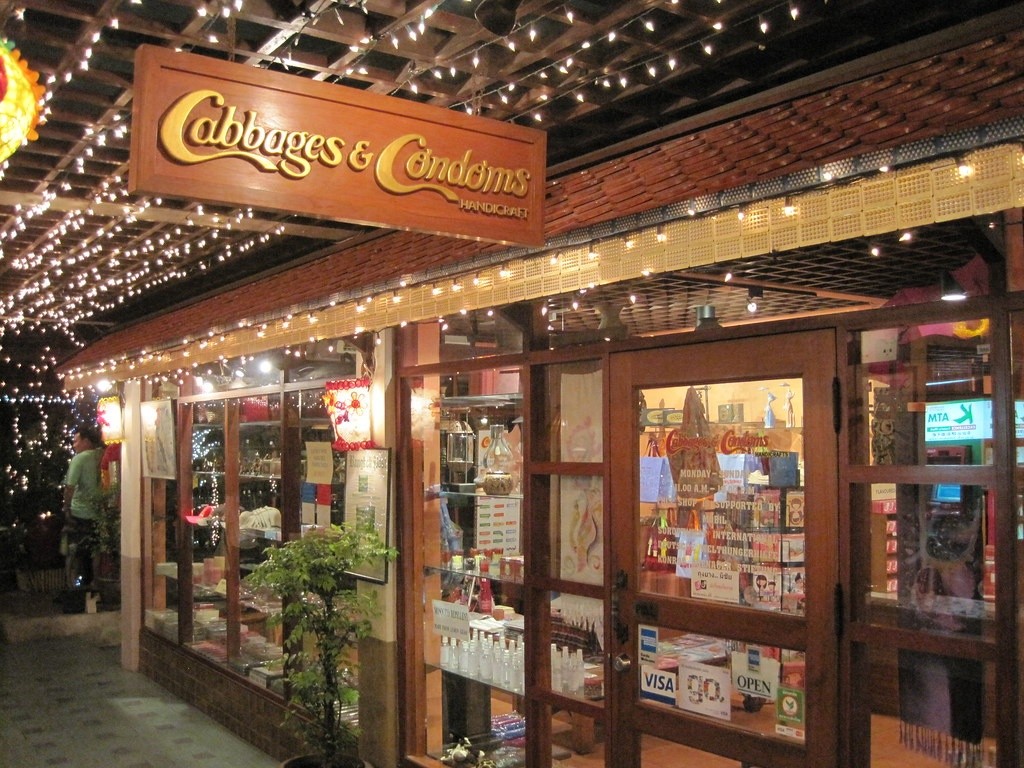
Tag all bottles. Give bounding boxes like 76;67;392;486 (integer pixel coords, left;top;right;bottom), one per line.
484;425;516;495
442;630;584;695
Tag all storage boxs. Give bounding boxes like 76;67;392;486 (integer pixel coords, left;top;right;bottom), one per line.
248;665;291;690
144;600;250;642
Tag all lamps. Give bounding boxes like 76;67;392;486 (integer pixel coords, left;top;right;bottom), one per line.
322;374;377;453
96;396;126;446
696;303;715;331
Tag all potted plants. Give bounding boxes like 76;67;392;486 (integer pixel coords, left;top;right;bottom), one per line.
237;522;398;768
78;485;123;611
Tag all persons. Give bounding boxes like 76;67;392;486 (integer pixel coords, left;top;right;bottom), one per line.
62;426;106;589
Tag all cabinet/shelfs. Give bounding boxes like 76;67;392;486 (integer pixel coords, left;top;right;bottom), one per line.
396;364;609;768
140;342;360;737
